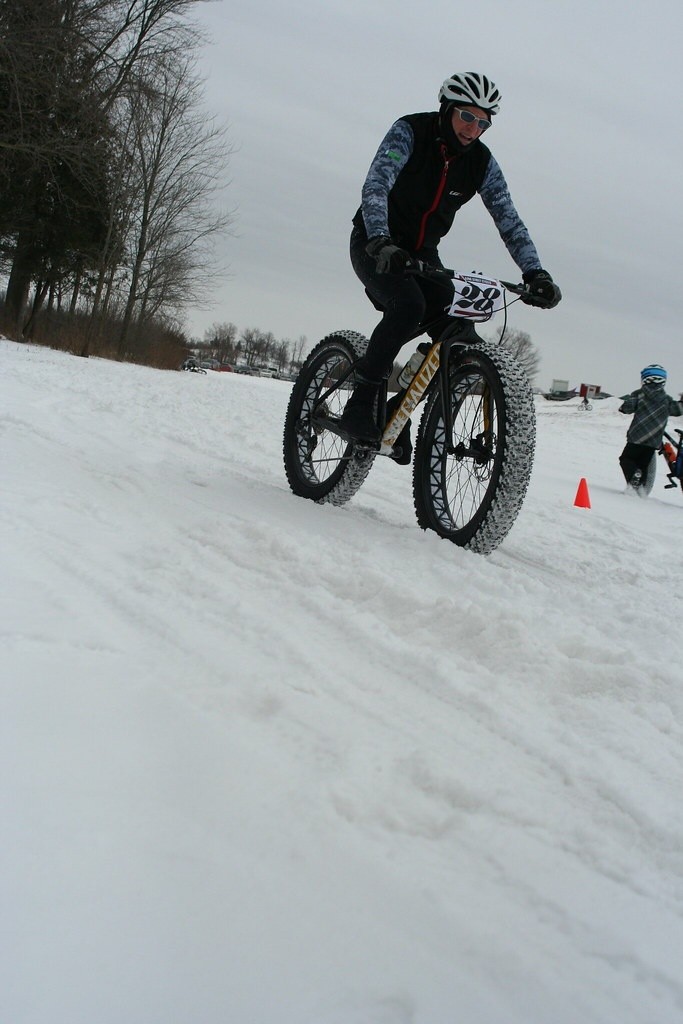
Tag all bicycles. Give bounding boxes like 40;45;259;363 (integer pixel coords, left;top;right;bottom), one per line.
578;402;593;411
624;398;683;498
284;267;555;555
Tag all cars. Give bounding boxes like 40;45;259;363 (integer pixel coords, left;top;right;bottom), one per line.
182;356;199;368
201;359;299;381
541;388;577;401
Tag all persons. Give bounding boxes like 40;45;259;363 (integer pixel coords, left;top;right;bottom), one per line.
582;395;589;410
619;365;683;491
342;71;559;464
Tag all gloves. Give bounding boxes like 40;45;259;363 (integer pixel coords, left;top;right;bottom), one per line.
366;237;413;283
520;269;562;309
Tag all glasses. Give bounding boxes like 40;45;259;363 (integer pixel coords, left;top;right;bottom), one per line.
453;107;492;131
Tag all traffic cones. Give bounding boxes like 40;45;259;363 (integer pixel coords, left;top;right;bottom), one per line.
572;477;590;510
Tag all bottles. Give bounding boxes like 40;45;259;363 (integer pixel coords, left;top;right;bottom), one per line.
399;342;431;389
665;442;676;462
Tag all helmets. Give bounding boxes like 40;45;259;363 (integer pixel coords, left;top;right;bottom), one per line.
640;364;667;387
437;72;502;115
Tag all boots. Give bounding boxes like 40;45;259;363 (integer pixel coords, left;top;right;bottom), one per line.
384;395;412;464
341;383;387;442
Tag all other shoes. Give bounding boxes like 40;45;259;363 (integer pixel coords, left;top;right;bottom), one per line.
630;469;643;490
